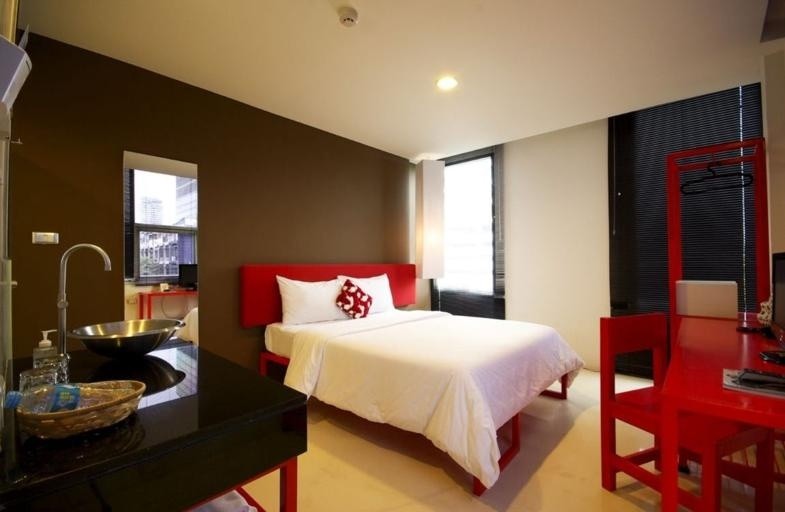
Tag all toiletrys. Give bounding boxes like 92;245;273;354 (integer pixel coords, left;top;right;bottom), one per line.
33;329;59;373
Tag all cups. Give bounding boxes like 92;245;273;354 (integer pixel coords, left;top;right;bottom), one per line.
31;351;72;385
17;367;59;392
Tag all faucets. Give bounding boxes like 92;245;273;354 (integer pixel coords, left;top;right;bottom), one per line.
58;241;112;352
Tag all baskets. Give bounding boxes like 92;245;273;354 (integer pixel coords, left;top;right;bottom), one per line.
14;379;145;441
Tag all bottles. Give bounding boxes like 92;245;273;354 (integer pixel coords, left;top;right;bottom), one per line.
4;383;117;414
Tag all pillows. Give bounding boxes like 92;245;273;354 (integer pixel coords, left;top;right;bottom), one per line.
275;272;397;325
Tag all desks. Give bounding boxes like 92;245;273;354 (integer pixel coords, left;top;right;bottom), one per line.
660;312;785;512
137;289;197;319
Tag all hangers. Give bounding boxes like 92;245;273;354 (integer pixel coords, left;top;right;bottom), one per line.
680;162;754;195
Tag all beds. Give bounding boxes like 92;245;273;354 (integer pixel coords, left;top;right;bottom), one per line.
237;263;569;496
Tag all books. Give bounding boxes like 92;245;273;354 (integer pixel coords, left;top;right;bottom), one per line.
722;366;785;399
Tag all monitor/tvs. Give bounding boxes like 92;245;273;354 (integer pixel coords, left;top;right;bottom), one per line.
771;250;785;327
179;264;197;290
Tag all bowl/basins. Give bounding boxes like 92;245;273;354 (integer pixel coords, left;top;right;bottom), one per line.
90;354;188;399
65;318;188;359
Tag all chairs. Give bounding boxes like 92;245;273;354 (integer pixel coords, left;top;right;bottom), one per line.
599;312;775;511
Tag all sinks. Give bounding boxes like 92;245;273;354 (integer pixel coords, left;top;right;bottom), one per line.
66;309;183;359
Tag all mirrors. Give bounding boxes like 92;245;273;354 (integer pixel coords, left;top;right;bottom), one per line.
120;149;199;346
